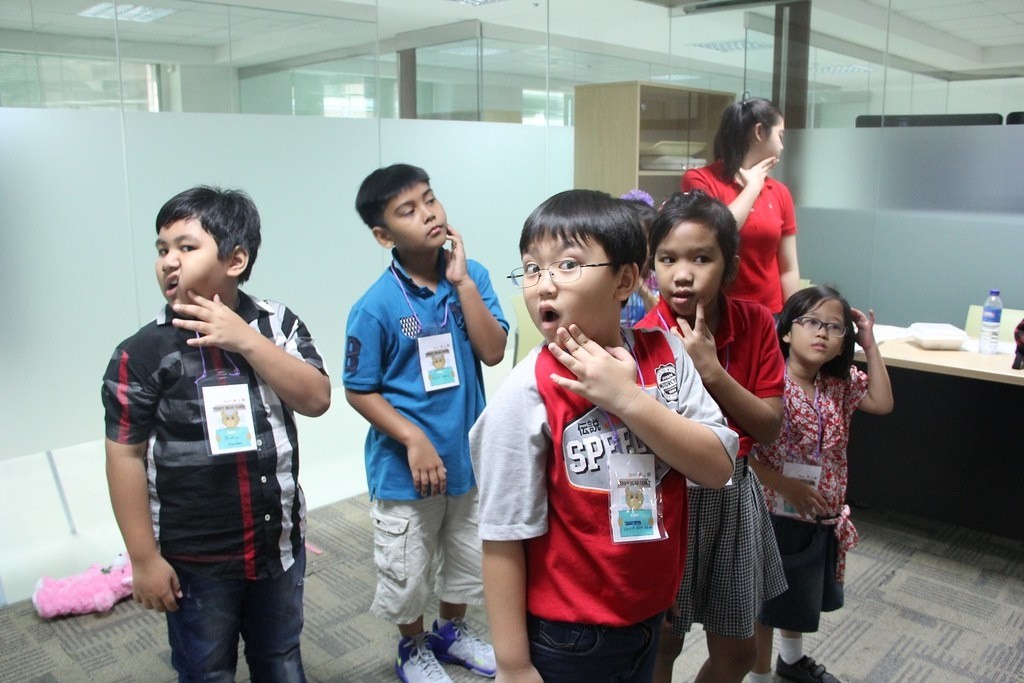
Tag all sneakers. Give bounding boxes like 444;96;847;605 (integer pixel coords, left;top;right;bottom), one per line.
430;620;496;677
777;653;840;683
395;631;453;683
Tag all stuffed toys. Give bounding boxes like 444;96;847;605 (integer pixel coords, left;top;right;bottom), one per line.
32;553;133;618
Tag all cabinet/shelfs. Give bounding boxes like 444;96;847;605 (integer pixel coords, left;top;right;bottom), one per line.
573;81;735;212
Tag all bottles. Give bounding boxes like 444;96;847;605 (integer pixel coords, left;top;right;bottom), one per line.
978;289;1002;357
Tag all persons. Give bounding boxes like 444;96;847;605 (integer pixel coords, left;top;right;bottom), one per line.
681;98;800;328
749;286;894;683
653;188;788;683
101;187;332;683
620;190;660;328
343;163;510;683
469;190;740;683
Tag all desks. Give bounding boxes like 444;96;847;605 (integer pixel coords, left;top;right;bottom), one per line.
851;322;1024;542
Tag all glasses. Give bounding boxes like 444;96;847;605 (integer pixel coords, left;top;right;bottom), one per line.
791;317;849;337
508;259;617;288
